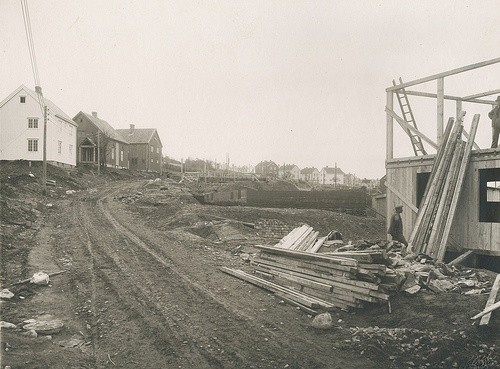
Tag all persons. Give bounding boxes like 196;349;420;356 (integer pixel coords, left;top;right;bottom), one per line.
387;204;408;246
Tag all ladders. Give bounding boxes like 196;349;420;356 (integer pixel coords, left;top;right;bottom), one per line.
392;77;427;158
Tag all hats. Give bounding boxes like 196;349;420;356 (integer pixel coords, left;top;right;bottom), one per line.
495;96;500;101
395;204;403;209
